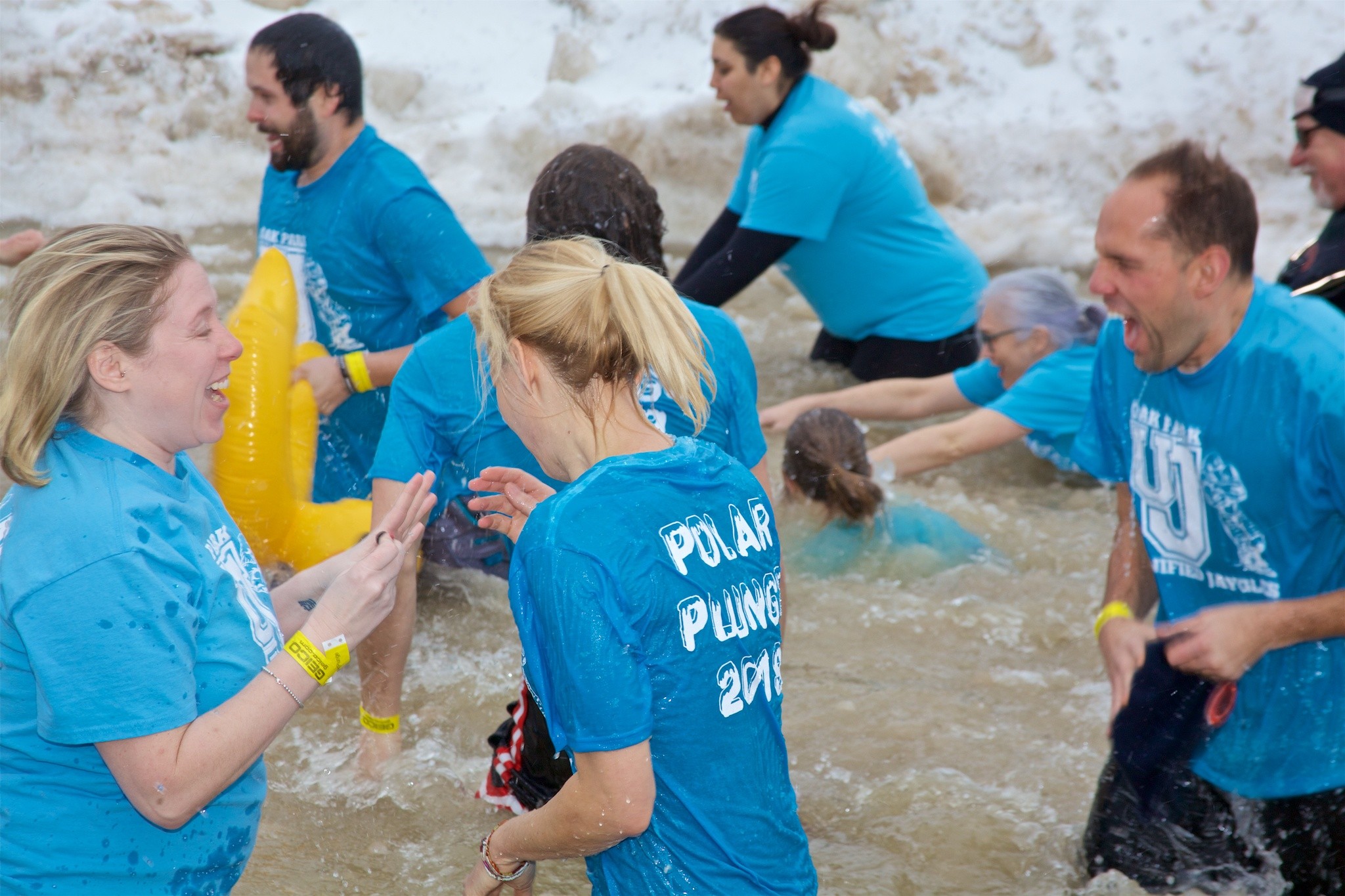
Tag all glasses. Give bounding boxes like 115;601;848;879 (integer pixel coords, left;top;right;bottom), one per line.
1292;123;1325;150
976;326;1021;356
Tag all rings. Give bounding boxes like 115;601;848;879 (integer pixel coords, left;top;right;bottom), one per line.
376;530;385;544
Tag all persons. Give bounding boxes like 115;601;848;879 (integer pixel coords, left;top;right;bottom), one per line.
0;225;438;896
762;269;1114;485
357;142;772;765
246;6;492;626
781;407;985;572
336;347;375;396
467;237;816;896
1271;51;1345;314
1083;135;1345;896
668;5;991;385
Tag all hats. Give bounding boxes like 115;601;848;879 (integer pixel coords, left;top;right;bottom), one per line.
1292;54;1345;137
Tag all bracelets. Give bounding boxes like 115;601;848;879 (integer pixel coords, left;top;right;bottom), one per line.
481;821;528;881
1095;600;1132;638
260;666;303;709
285;629;350;686
360;703;400;735
